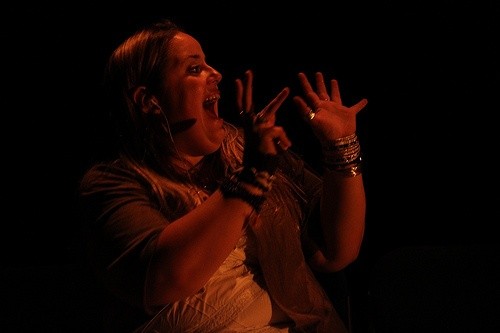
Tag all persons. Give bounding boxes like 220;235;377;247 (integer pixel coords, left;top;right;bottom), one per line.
77;23;372;333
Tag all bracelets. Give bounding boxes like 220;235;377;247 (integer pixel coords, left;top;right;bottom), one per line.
220;153;277;213
326;133;363;177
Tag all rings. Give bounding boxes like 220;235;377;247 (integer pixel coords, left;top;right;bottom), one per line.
320;97;330;101
304;109;316;121
257;112;264;120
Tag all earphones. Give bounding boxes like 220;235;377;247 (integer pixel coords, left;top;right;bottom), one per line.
150;97;159;109
